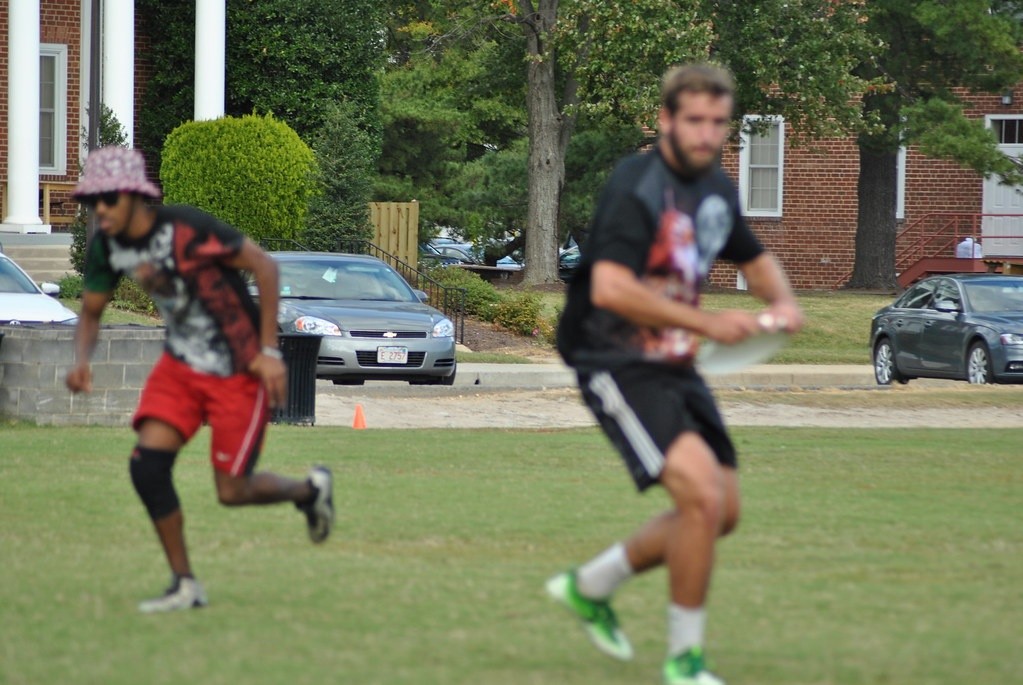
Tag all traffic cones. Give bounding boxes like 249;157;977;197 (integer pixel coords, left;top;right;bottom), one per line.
352;404;365;430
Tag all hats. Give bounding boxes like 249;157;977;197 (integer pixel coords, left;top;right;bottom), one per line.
70;145;162;204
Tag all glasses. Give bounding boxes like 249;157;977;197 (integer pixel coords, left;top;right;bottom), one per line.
83;192;121;208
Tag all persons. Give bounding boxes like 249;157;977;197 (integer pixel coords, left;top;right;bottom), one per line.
65;146;334;614
547;61;804;685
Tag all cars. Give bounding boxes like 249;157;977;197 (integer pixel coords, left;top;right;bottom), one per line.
424;230;580;269
244;248;458;387
869;272;1023;386
0;251;80;328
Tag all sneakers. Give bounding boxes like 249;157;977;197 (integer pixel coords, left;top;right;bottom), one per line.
541;560;632;662
661;645;726;685
137;575;205;615
295;466;334;542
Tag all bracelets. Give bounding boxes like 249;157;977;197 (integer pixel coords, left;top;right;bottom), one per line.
261;345;283;360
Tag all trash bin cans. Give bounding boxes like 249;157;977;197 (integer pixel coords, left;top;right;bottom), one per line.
271;333;325;427
